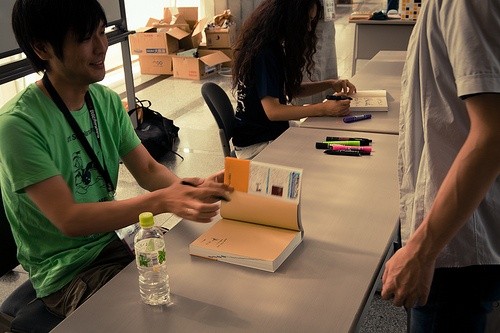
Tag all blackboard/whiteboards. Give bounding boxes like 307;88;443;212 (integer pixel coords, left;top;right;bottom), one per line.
0;0;136;85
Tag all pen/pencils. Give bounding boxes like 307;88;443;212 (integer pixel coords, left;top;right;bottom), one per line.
326;95;353;100
316;136;375;156
182;181;229;202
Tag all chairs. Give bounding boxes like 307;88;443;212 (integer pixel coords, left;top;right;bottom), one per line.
201;83;237;160
0;186;66;333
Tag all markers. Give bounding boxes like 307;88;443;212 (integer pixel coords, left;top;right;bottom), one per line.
343;114;371;123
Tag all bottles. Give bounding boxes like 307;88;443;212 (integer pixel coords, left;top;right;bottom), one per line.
133;212;171;306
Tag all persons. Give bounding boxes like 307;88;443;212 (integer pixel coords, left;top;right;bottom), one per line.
0;0;234;319
381;0;500;333
232;0;356;161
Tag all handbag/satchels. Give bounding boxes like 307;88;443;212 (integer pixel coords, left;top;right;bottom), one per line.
121;96;179;160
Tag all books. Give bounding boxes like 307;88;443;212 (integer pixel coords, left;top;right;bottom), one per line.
347;89;388;111
189;157;304;272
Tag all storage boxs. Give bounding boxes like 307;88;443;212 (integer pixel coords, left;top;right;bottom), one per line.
129;7;236;81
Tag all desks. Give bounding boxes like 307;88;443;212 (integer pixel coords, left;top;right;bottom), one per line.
46;126;400;333
300;51;407;135
348;18;417;78
